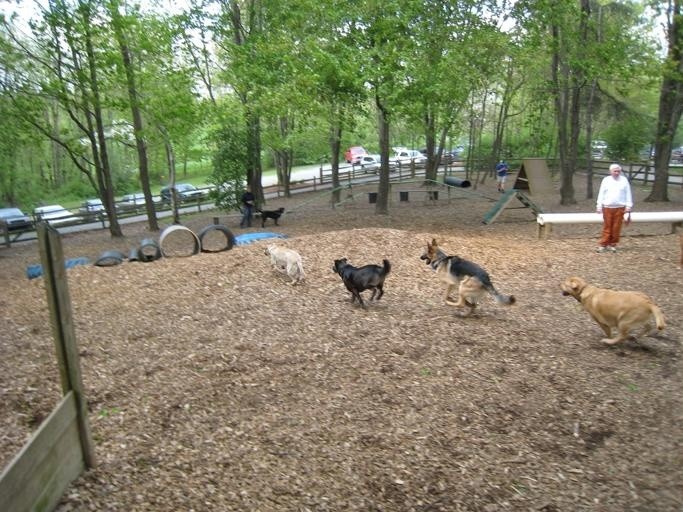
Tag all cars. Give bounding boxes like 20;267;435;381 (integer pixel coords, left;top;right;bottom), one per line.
640;144;682;162
593;139;611;154
160;183;203;204
79;198;116;218
118;194;150;211
344;145;467;176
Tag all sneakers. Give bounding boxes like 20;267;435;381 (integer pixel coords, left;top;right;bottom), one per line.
595;245;617;254
497;188;506;194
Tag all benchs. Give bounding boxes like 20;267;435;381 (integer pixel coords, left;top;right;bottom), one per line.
533;212;682;244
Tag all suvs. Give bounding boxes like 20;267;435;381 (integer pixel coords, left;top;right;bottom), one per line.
0;207;30;231
32;206;78;226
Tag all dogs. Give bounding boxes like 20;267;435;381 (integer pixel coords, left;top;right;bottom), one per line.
333;258;390;308
421;239;515;318
558;277;666;346
255;208;285;228
262;243;306;286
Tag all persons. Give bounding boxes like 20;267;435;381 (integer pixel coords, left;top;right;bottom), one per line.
239;184;256;227
595;164;632;254
495;159;510;193
